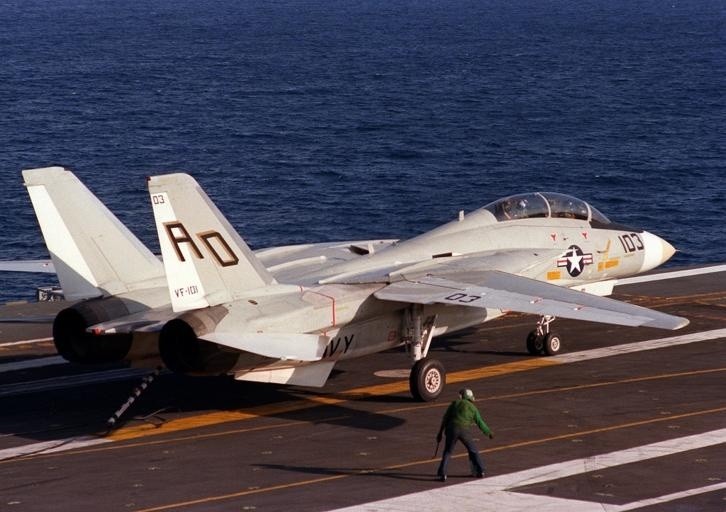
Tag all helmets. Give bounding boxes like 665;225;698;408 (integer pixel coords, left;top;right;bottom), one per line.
459;389;473;401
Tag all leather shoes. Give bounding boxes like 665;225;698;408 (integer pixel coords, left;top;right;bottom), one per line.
440;475;446;481
477;473;485;477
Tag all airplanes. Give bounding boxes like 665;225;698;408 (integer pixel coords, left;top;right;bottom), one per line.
0;164;695;407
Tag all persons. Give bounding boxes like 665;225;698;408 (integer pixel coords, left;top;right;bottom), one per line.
434;388;495;483
559;200;577;219
514;198;531;219
